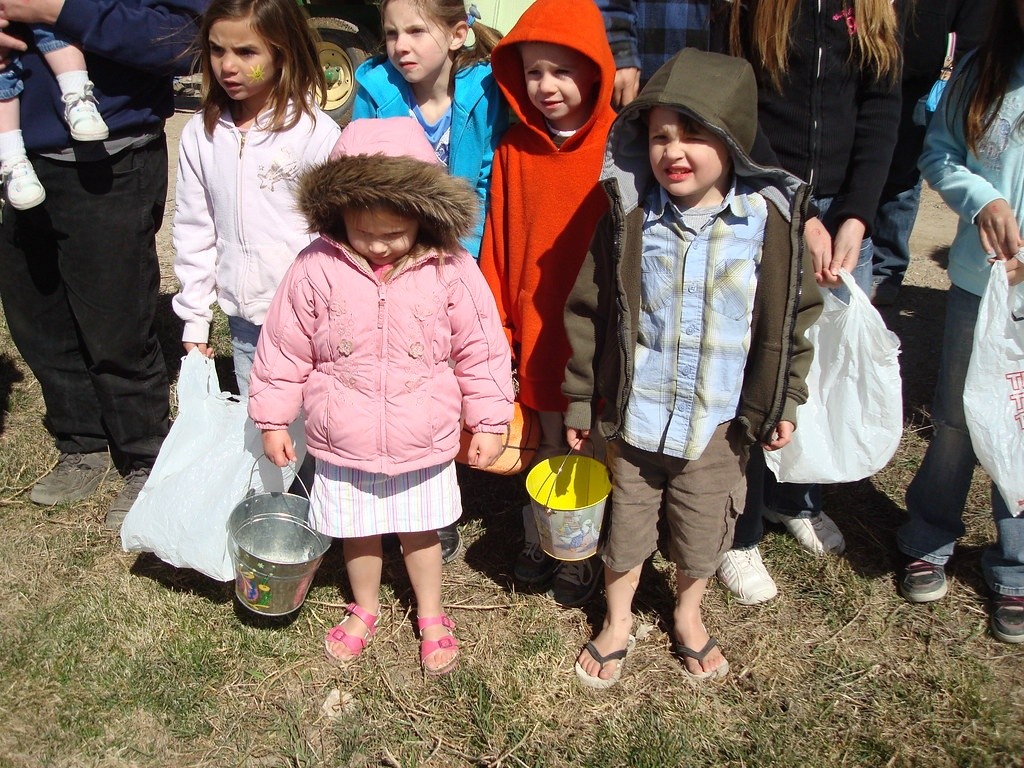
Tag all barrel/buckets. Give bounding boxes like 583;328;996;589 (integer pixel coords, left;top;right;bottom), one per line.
226;452;333;616
525;438;614;561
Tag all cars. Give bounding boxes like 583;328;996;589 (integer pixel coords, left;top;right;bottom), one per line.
174;0;376;127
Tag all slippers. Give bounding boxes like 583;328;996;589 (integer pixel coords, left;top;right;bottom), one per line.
676;623;728;679
574;633;636;689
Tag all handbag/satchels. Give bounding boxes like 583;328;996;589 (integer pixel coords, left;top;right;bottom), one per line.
763;266;904;484
121;347;306;582
963;246;1024;517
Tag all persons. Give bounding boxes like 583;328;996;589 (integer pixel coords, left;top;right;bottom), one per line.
1;0;1024;643
246;117;513;676
558;48;824;690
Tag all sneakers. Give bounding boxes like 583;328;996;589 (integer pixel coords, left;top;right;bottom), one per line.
107;467;152;529
60;80;108;141
901;557;948;603
30;447;114;505
716;545;777;606
0;156;46;210
762;505;845;555
990;591;1024;642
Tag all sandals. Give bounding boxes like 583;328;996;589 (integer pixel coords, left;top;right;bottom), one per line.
324;602;381;662
416;609;459;675
